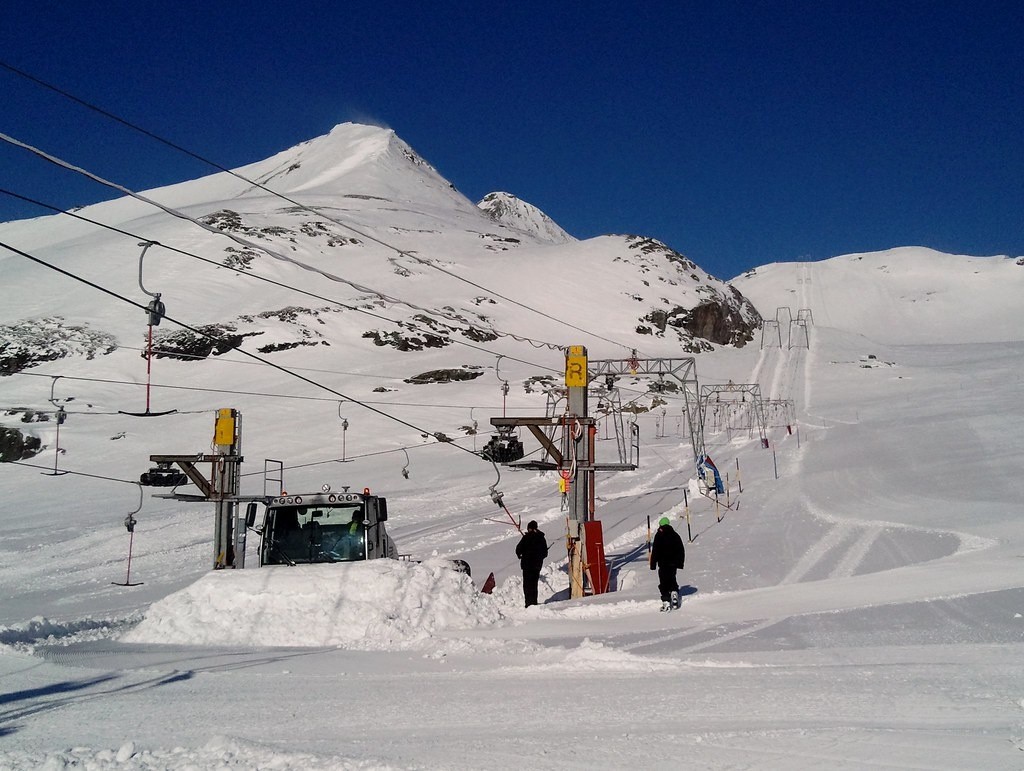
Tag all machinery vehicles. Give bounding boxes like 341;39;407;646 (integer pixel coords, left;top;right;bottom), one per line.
247;484;472;577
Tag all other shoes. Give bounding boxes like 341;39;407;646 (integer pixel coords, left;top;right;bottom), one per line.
660;601;670;611
671;598;678;609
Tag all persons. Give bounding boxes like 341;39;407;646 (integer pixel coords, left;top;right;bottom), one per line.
650;517;685;612
516;521;549;608
559;477;567;511
342;509;363;541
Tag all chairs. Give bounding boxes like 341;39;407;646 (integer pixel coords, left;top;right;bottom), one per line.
279;521;322;562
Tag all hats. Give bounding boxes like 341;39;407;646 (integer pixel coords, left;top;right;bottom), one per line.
528;521;538;529
659;517;670;526
